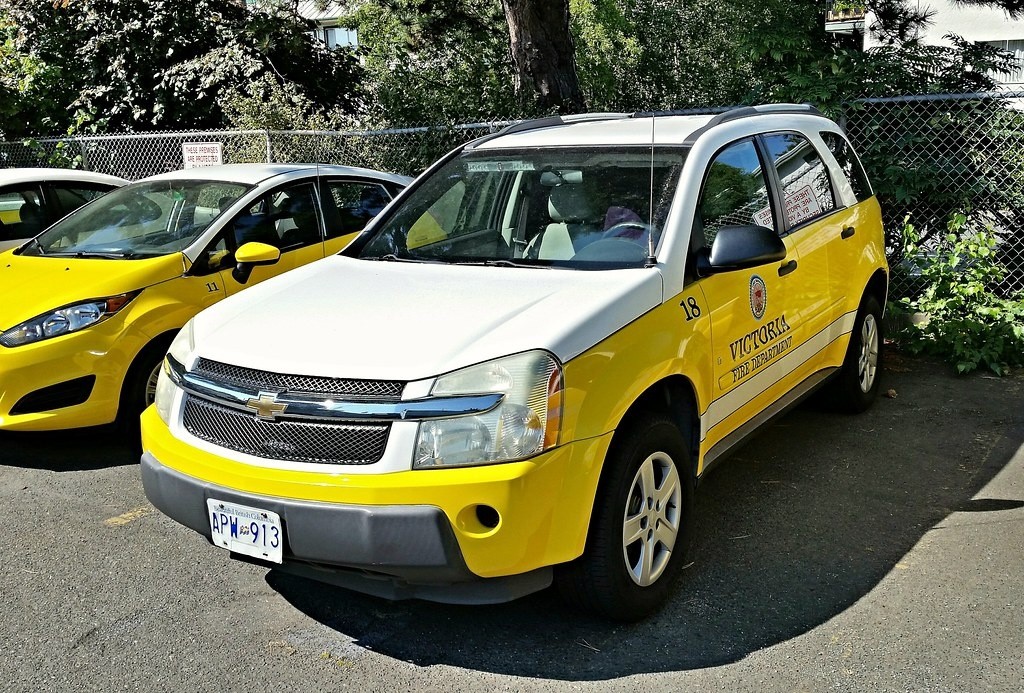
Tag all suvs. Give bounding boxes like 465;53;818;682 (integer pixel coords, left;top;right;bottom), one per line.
146;101;889;624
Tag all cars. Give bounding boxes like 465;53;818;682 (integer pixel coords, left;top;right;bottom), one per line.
0;165;453;431
0;168;220;254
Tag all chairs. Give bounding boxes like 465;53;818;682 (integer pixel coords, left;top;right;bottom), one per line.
219;196;255;253
538;174;613;260
20;202;41;222
342;188;381;228
